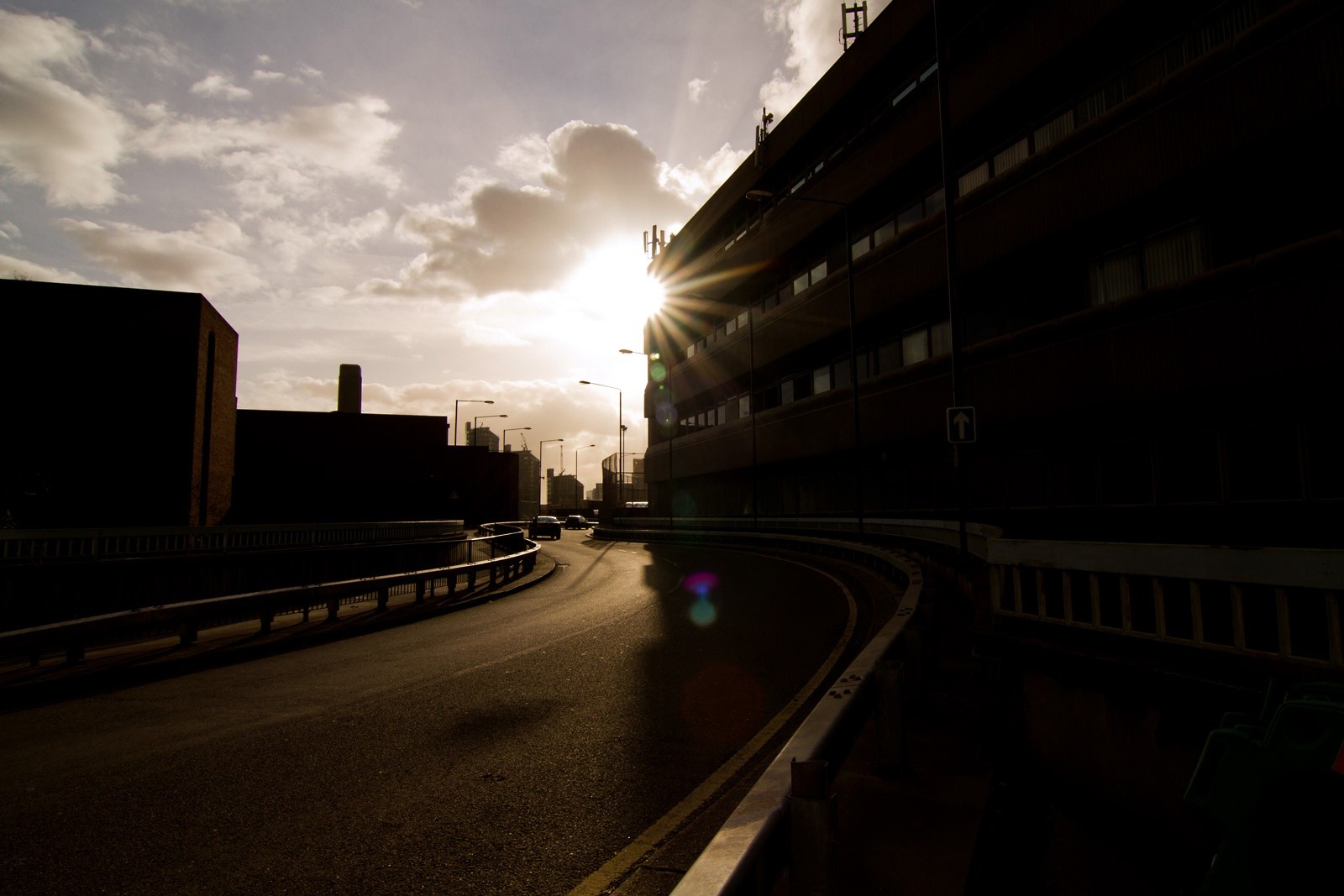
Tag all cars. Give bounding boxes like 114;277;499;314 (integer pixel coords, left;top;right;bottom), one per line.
528;516;561;539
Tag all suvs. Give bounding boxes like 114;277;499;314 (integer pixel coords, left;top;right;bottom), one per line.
564;515;589;529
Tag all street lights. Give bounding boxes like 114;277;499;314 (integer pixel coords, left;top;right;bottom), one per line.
620;349;673;517
688;291;757;478
539;439;563;516
503;427;531;452
580;381;622;496
454;400;494;446
619;453;635;500
474;415;508;446
575;445;595;514
